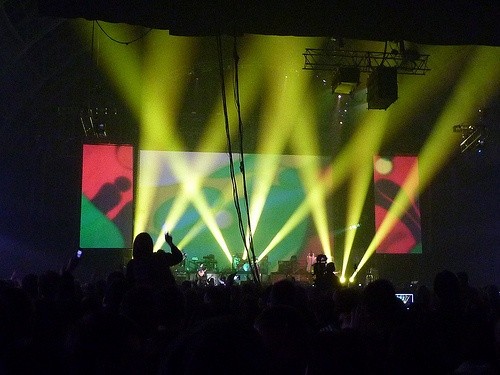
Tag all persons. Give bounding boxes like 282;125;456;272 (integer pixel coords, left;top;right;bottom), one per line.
125;231;184;293
0;249;500;374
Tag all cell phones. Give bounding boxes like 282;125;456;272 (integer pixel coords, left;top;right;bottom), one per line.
76;248;83;259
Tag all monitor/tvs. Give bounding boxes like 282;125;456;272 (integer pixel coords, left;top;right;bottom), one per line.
394;294;414;310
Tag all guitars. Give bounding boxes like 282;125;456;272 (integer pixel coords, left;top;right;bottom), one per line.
197;269;207;276
